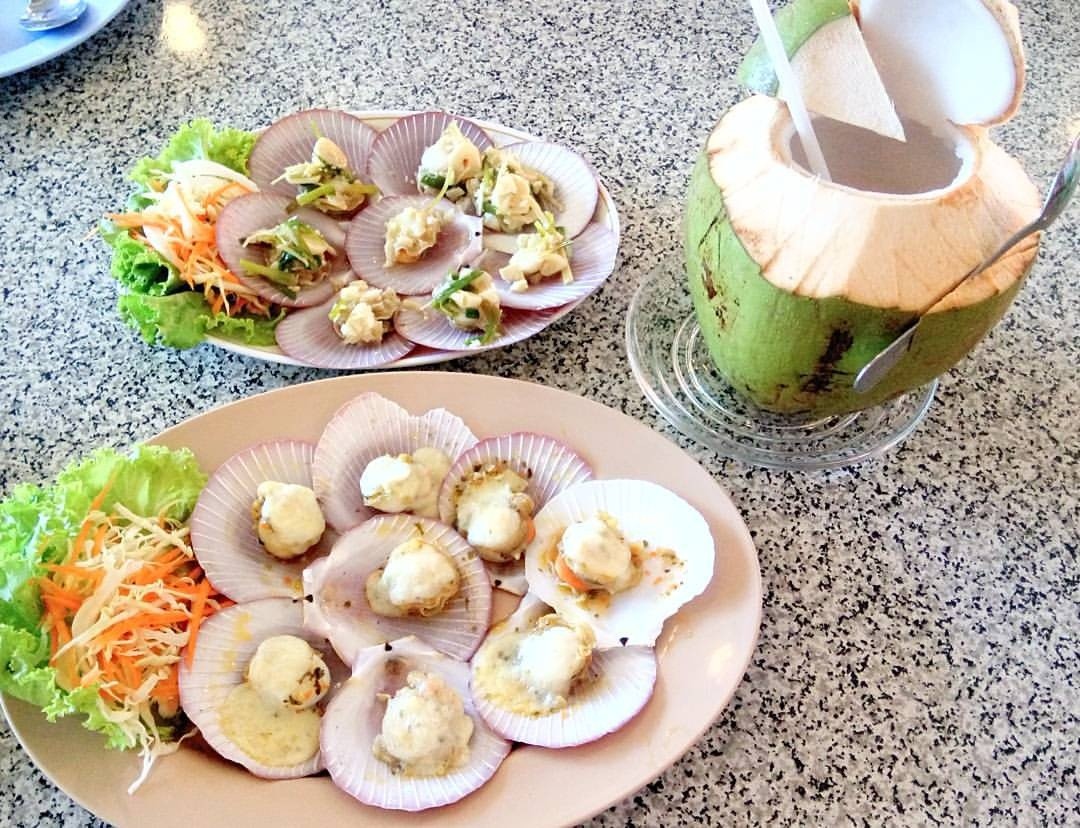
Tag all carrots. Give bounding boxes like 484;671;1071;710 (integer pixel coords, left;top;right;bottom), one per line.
31;483;238;718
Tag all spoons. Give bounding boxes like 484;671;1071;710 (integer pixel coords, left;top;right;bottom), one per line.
855;136;1080;390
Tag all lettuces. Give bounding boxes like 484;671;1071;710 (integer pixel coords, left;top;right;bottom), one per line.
95;115;290;352
0;443;208;748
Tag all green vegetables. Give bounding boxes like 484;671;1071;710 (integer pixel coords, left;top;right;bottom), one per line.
239;161;564;346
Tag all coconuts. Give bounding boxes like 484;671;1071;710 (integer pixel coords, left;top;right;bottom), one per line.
678;0;1044;413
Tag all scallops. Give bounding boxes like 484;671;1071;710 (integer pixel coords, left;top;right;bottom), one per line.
176;110;715;813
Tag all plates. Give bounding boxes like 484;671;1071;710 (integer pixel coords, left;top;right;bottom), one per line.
0;371;764;828
625;248;939;470
0;0;130;77
204;110;621;370
